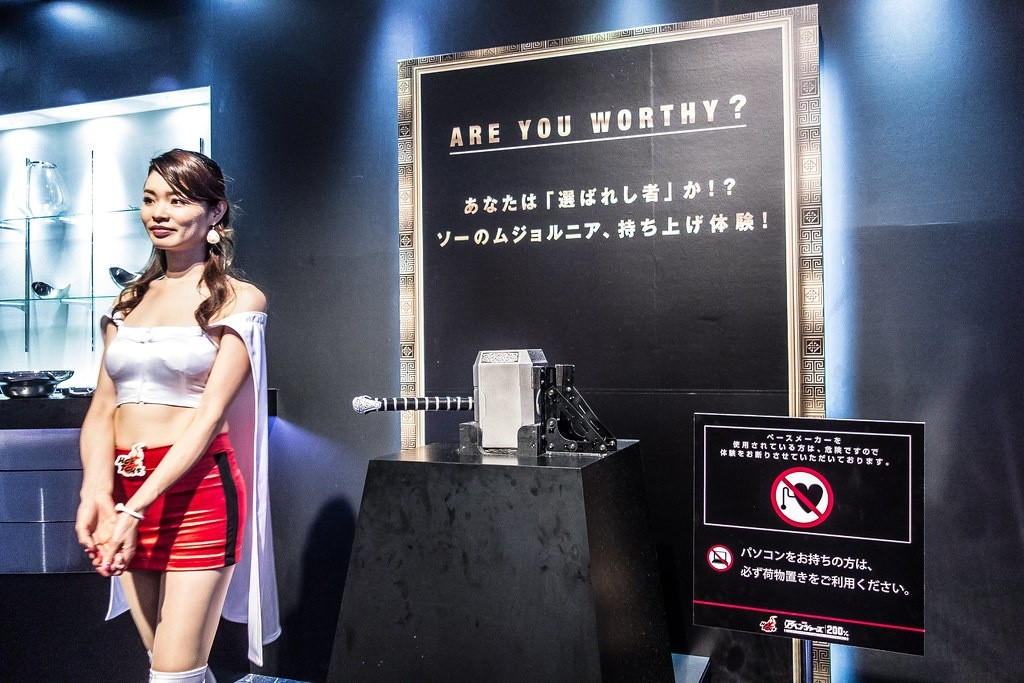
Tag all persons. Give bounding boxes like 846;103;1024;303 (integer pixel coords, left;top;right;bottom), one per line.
75;149;268;683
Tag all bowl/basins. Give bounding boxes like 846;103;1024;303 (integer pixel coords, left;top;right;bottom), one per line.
0;384;56;398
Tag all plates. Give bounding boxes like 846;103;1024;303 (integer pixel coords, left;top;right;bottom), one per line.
0;370;74;381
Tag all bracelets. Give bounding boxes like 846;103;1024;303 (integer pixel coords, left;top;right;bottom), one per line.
114;503;145;521
